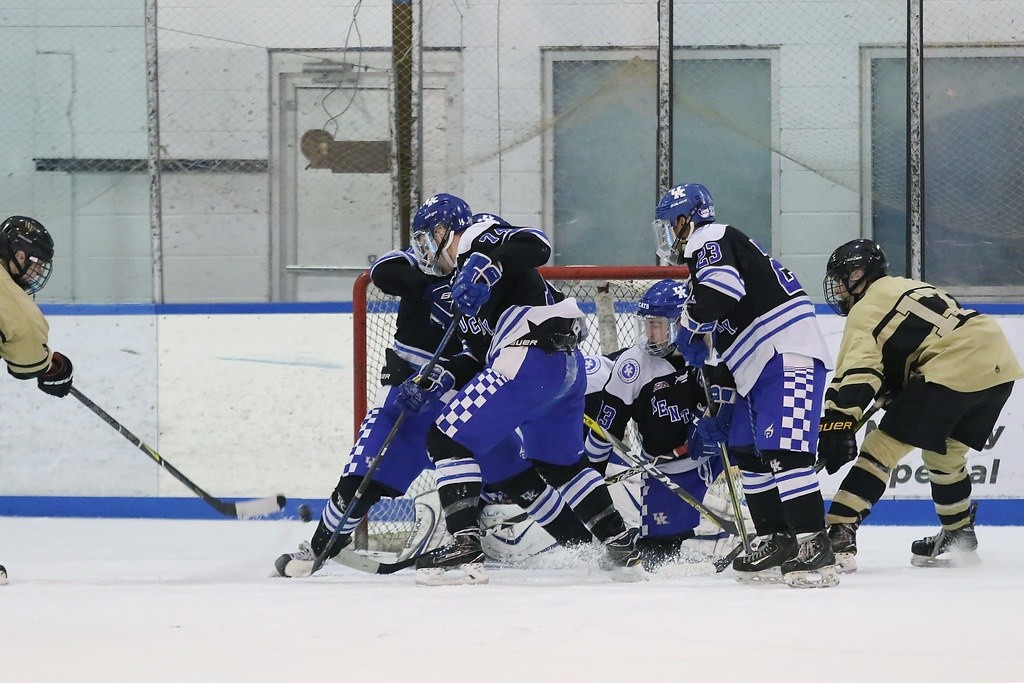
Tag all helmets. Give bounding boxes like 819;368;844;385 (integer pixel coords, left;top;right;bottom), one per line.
0;216;54;296
822;238;887;316
410;193;473;277
651;184;716;268
634;279;690;358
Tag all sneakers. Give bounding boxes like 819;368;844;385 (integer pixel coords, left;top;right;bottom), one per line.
413;530;489;585
599;524;647;582
780;526;839;588
730;533;799;580
910;523;979;566
274;546;325;578
827;522;857;574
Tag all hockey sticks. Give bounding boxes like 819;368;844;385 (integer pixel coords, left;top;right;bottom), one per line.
674;395;882;576
582;413;759;537
699;365;754;556
331;443;690;576
68;384;286;519
284;310;465;578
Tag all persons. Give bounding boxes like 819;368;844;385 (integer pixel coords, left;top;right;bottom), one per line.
269;184;841;589
0;216;73;585
818;238;1024;574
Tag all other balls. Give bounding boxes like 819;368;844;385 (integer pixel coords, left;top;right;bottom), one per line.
299;503;313;522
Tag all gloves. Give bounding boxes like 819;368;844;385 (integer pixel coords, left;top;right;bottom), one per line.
394;365;456;419
817;409;858;475
875;381;900;411
450;252;502;316
697;385;737;442
677;327;709;368
37;351;72;398
